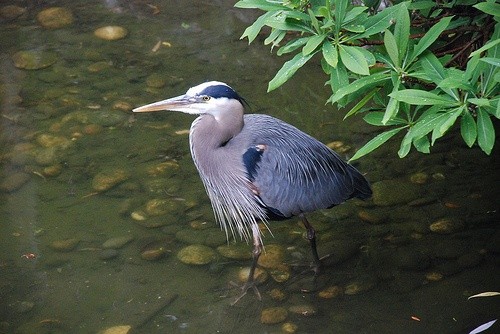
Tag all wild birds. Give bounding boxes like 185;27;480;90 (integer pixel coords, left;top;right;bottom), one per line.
132;81;374;307
